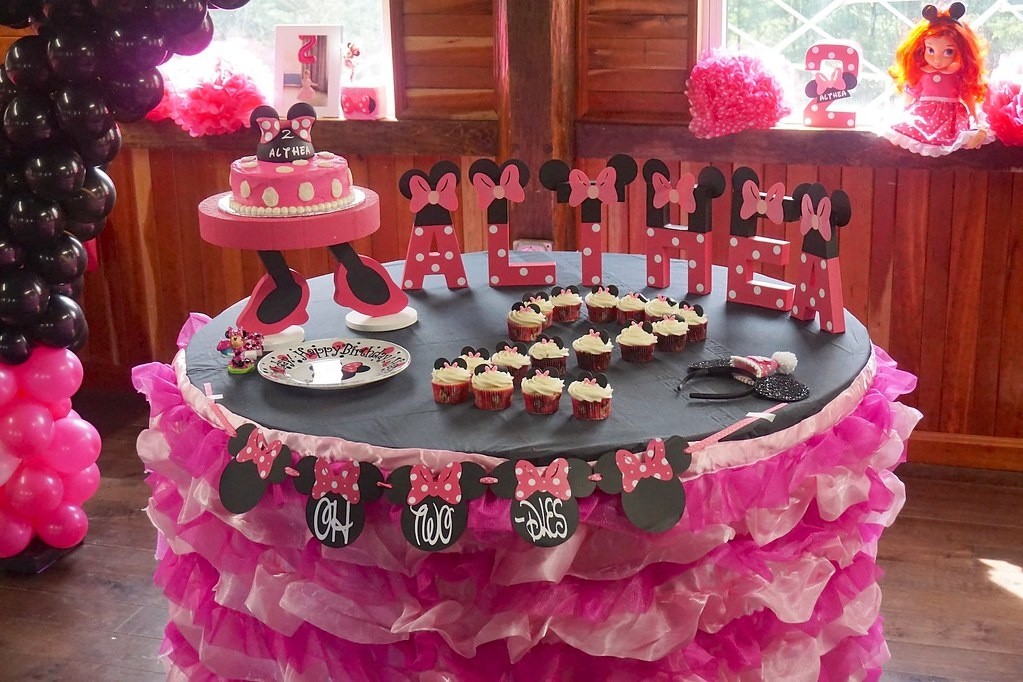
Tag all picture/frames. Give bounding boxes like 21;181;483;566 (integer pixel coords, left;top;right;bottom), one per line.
275;24;339;117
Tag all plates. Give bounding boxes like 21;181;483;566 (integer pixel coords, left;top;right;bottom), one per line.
256;337;412;390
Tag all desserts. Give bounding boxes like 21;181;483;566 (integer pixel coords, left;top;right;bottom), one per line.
431;286;709;421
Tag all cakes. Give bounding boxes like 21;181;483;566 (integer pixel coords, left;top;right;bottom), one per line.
229;105;355;214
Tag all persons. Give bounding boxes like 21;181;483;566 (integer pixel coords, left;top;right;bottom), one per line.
873;1;996;156
297;70;318;102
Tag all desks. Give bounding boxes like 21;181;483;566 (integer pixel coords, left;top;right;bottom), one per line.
134;247;926;682
